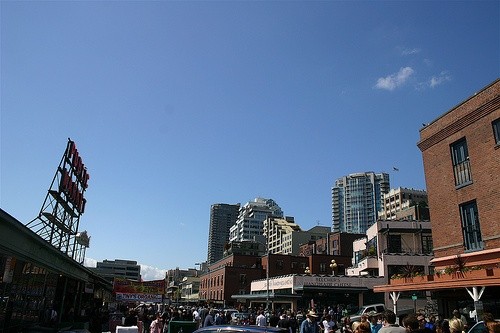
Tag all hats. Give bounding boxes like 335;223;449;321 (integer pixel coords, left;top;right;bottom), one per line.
367;311;382;316
306;312;319;317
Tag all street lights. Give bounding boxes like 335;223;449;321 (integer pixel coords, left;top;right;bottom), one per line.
194;262;202;276
255;241;270;310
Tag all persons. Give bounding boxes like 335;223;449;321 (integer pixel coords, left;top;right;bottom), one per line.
123;301;500;333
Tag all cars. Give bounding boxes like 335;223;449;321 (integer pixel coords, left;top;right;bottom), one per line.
191;324;290;333
463;319;500;333
214;308;256;325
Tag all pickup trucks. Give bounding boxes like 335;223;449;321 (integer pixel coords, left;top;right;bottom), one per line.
340;303;413;326
